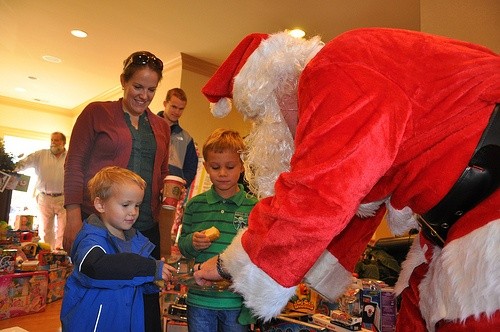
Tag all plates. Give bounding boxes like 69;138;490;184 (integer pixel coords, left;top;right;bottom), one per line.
188;279;231;291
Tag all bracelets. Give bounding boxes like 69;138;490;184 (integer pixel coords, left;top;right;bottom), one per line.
217;255;232;280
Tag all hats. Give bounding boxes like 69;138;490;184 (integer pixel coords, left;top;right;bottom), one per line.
201;32;303;118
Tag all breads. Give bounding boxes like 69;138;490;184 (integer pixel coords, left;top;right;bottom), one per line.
202;226;220;241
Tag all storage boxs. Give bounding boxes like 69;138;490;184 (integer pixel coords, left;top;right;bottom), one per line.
47;265;74;304
0;270;48;321
15;215;33;230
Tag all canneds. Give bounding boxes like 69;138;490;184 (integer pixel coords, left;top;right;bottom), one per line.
19;215;33;231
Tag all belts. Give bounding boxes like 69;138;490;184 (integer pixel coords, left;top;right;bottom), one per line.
416;102;499;248
41;192;62;197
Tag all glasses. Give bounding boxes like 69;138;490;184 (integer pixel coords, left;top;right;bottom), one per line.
123;54;163;75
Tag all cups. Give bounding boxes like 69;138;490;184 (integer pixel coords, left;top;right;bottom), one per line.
162;175;183;209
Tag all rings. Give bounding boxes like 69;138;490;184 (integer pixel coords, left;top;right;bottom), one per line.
197;263;203;269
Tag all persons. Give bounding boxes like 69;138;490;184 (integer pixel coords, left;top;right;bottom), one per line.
192;27;500;332
11;50;259;332
